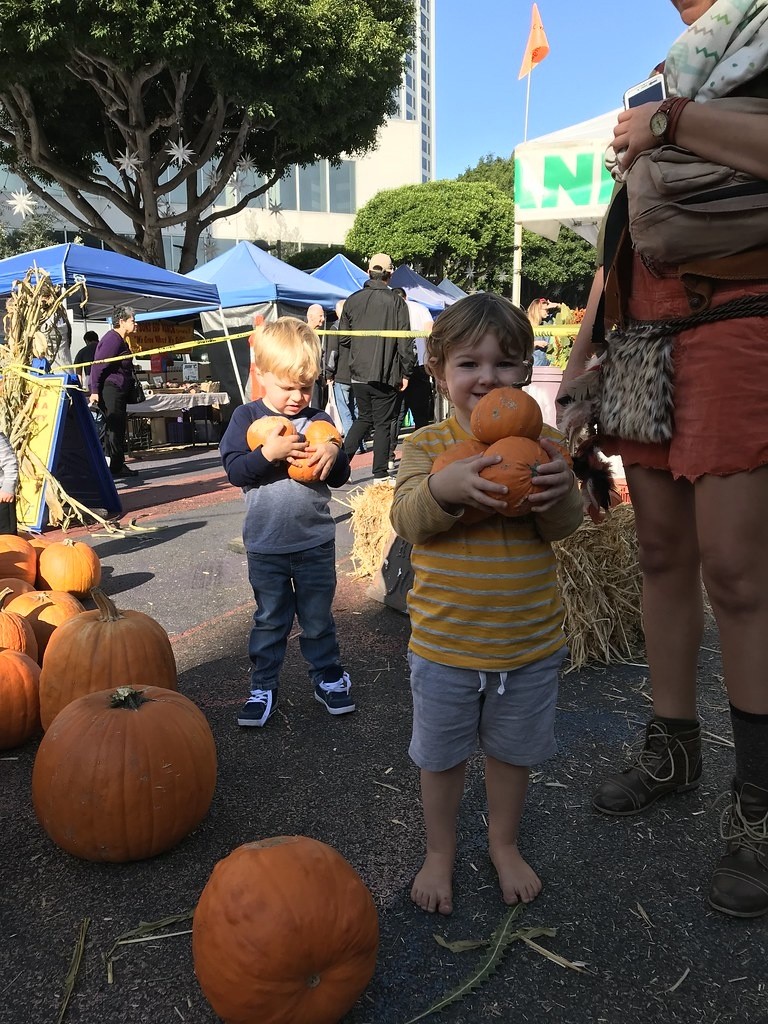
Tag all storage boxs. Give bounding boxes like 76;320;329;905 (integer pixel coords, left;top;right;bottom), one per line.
183;363;212;382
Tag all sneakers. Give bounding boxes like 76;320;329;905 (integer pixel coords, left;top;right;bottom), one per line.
315;671;355;714
592;720;703;817
708;776;768;918
237;687;278;726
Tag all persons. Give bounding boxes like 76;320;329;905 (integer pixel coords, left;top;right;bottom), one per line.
526;297;571;366
306;304;328;411
390;292;587;910
226;316;356;726
325;299;367;455
89;306;138;476
0;431;20;536
328;254;416;484
555;0;767;917
388;287;436;469
74;331;100;389
31;351;54;375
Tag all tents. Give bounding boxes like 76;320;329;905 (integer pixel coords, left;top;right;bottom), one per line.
388;265;459;321
0;241;246;405
309;254;444;321
439;277;470;300
183;242;355;440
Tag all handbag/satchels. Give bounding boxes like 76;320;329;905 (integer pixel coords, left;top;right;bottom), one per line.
624;96;768;276
601;321;673;441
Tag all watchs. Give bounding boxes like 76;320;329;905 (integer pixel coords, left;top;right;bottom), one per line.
650;92;680;145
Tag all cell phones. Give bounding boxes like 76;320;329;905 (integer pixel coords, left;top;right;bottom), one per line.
624;73;667;110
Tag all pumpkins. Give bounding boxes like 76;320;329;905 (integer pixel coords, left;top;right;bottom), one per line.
31;684;217;863
0;529;176;751
428;380;574;522
247;415;342;483
192;835;379;1024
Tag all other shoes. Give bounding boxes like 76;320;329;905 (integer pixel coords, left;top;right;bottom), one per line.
372;472;394;481
111;463;138;477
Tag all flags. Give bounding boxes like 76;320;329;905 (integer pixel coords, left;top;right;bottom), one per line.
518;3;549;80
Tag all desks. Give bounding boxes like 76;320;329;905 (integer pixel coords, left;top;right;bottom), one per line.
84;392;231;458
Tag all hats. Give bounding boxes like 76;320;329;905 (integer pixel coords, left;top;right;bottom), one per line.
369;253;393;273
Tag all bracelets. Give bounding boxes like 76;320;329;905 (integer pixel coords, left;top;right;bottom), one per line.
333;443;340;451
665;96;696;145
